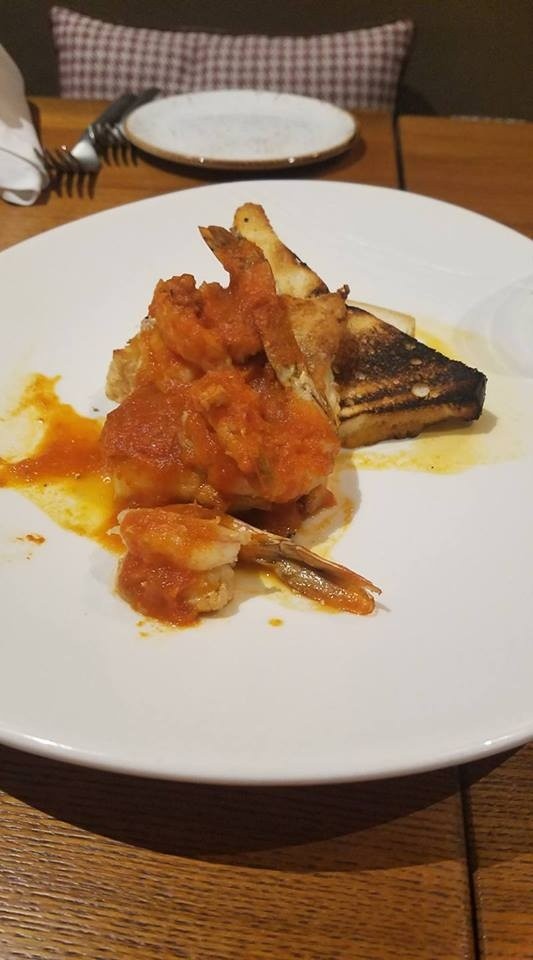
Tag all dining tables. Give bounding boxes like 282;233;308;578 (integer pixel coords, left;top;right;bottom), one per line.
0;84;532;958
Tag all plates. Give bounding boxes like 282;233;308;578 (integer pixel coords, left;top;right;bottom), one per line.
0;178;533;785
122;86;358;170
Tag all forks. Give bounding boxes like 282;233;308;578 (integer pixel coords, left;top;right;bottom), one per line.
88;87;164;150
33;90;131;174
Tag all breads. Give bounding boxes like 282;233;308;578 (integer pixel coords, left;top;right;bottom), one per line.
233;195;487;441
